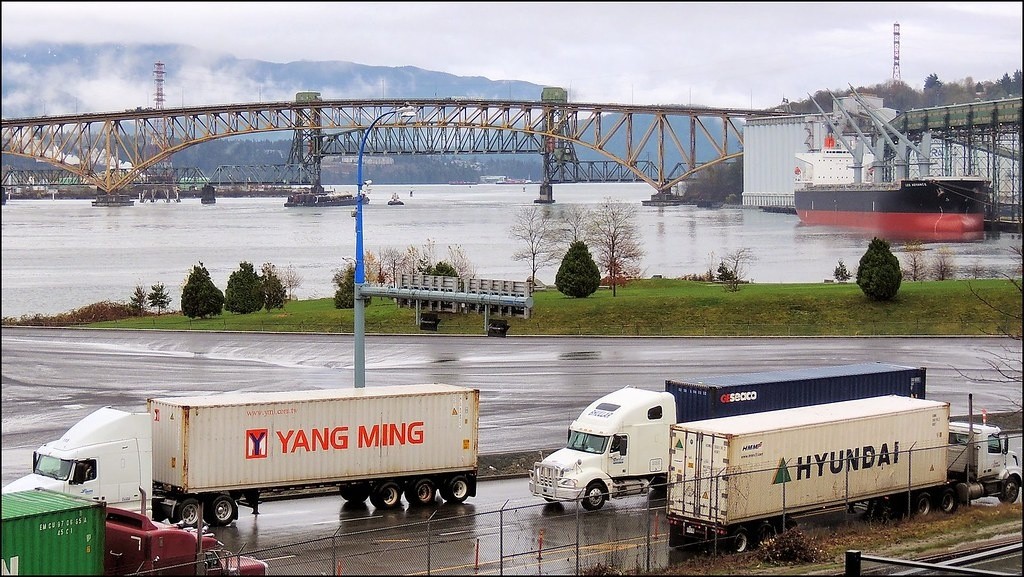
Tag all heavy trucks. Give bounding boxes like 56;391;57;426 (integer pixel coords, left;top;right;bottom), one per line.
664;393;1021;552
1;383;489;529
530;362;930;510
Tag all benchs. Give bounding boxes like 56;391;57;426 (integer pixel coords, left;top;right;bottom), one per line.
598;286;610;289
533;286;545;292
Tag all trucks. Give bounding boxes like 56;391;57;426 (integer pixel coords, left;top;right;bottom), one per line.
0;488;272;576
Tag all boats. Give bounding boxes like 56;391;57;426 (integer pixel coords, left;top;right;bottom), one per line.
794;83;993;243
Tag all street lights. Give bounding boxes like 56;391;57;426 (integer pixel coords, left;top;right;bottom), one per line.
352;107;417;390
